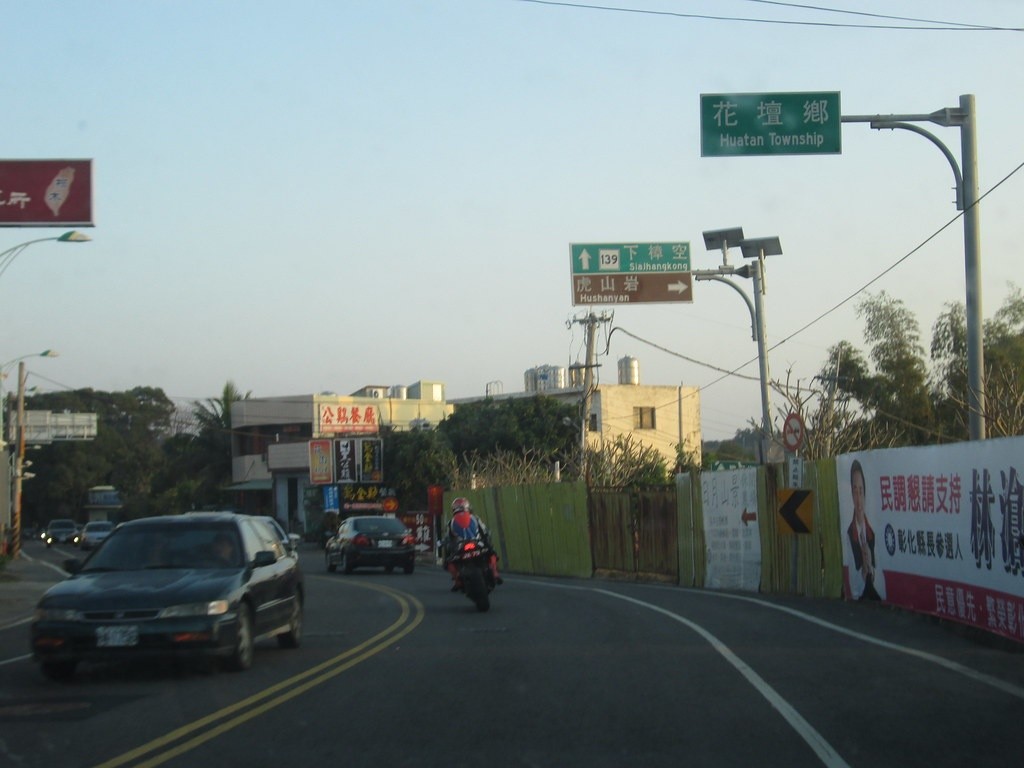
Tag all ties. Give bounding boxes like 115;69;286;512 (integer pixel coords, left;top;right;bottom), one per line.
859;524;867;546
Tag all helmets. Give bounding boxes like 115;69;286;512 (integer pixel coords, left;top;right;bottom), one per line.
452;498;471;515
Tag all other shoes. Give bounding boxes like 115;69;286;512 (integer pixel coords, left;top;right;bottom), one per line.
451;583;464;591
495;577;503;586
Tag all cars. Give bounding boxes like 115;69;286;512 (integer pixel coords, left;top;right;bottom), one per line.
41;518;114;551
325;515;417;576
31;511;305;675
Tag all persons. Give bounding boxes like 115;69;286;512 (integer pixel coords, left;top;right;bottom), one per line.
315;512;341;547
843;459;887;601
441;496;503;592
206;534;241;567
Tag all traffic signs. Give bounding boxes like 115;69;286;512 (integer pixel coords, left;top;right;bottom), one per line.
569;241;691;273
570;273;693;306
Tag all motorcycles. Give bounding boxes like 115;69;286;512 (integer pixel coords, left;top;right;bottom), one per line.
436;532;503;612
